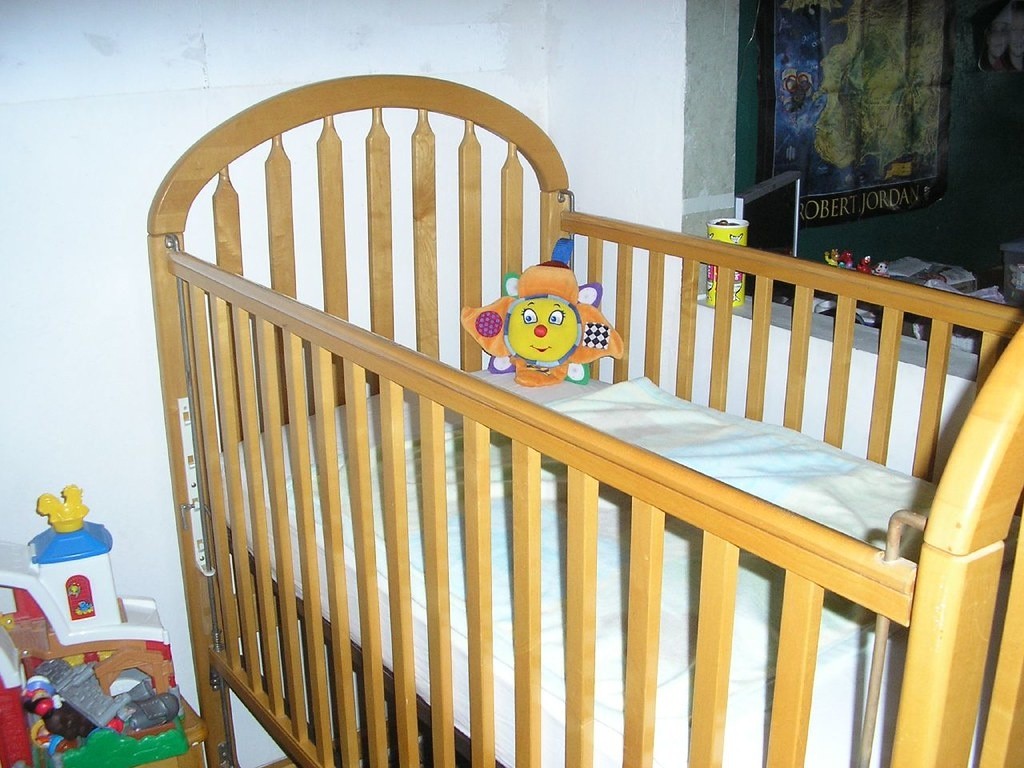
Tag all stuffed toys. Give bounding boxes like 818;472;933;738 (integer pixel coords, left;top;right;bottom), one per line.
461;238;624;387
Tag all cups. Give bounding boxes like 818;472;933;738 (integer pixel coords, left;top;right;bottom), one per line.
706;217;750;307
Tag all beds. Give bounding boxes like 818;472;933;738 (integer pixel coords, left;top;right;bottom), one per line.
147;73;1024;768
